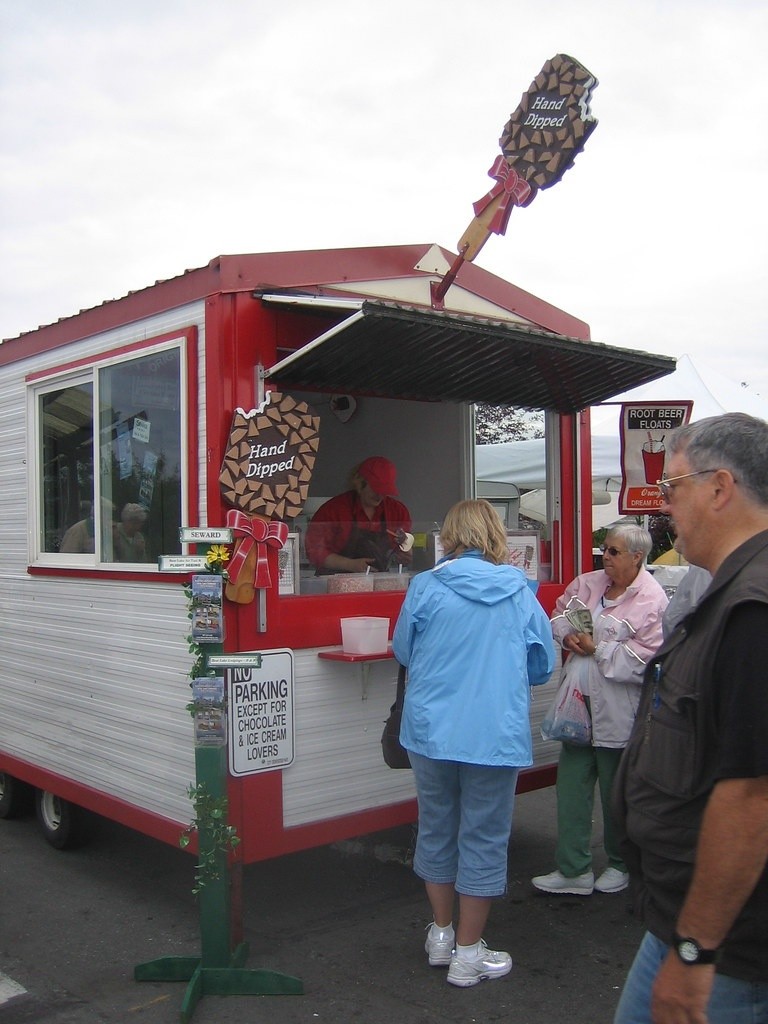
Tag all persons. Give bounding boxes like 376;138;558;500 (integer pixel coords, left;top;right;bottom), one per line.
60;497;116;553
113;503;147;563
304;456;414;577
531;412;768;1023
393;499;555;988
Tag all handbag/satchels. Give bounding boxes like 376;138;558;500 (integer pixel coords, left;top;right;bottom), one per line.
381;665;412;769
539;655;592;746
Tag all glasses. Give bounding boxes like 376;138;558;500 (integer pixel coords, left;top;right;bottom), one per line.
599;544;639;556
656;469;737;504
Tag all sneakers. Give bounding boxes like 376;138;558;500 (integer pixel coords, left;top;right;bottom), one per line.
424;922;455;965
531;869;595;896
447;938;513;987
595;867;629;893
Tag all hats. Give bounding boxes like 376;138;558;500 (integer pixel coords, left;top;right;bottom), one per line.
359;456;399;496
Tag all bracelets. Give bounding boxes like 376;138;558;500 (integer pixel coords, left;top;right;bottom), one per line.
592;645;597;654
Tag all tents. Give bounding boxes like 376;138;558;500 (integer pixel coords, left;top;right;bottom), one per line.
475;433;627;531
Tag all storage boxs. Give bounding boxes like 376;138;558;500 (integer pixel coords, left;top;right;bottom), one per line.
300;565;420;596
340;616;391;656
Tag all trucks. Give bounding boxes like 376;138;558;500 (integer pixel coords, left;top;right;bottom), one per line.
0;54;694;1024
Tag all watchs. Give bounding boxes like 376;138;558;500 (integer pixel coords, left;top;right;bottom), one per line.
670;930;728;965
